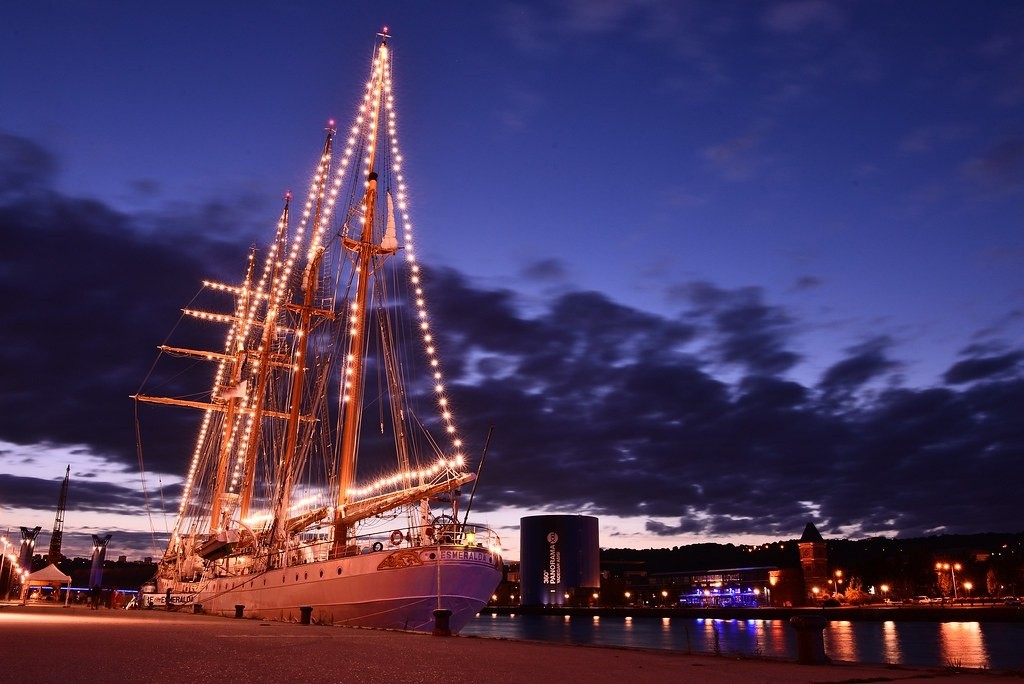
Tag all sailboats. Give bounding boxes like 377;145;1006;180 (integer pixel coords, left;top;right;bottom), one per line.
127;26;505;636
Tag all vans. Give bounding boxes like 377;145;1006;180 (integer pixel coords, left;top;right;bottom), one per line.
914;596;931;604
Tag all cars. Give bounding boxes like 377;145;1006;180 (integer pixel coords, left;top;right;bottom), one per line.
931;598;942;604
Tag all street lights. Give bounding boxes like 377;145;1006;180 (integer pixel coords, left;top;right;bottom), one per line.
0;528;30;584
944;562;962;599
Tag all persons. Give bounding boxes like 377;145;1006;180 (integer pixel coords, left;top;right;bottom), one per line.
25;579;174;614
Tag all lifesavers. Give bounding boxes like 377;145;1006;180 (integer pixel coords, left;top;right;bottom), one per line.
389;529;404;547
373;542;384;552
